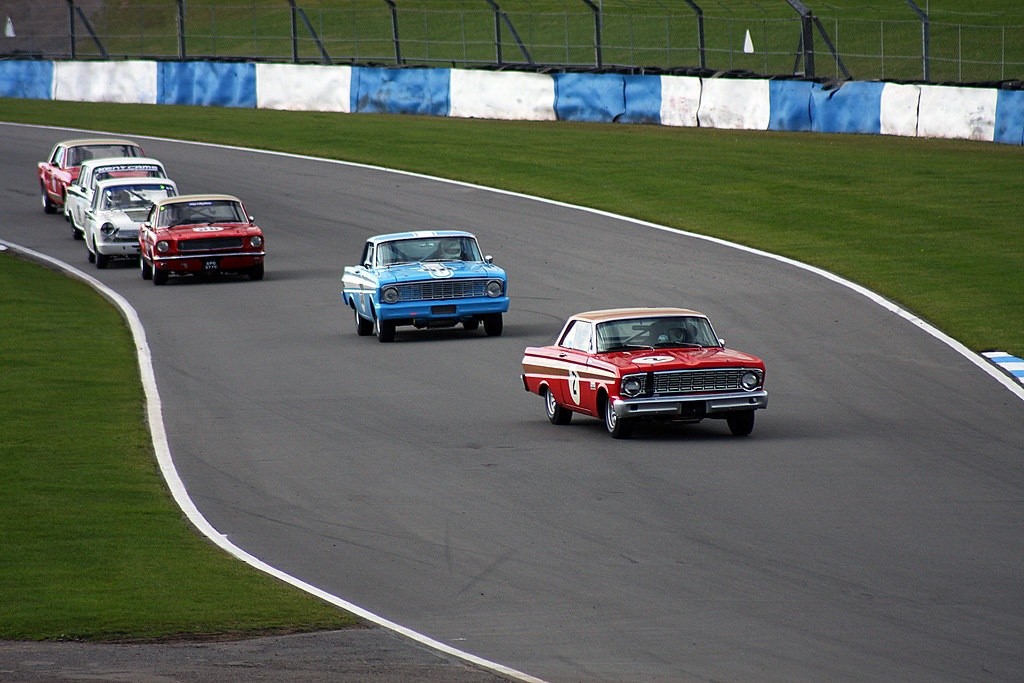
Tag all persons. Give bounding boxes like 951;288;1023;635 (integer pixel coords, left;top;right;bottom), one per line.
69;147;82;166
434;240;471;261
664;321;694;344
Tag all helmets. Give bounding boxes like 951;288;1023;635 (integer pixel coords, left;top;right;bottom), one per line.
664;319;686;342
439;239;461;259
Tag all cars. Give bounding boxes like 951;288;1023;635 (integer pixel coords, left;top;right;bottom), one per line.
66;156;171;242
342;231;511;343
520;308;769;435
138;195;266;285
36;139;150;217
86;176;188;270
